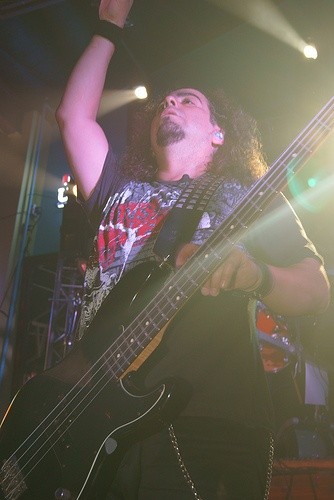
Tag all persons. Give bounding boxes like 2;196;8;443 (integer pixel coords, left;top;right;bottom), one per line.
56;1;332;500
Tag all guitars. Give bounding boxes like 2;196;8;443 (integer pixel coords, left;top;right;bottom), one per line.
2;94;334;499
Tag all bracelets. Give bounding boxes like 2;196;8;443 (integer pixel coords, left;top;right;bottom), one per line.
94;19;123;50
240;258;274;300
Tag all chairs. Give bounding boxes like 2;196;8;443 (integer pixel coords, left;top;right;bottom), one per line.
272;436;320;500
